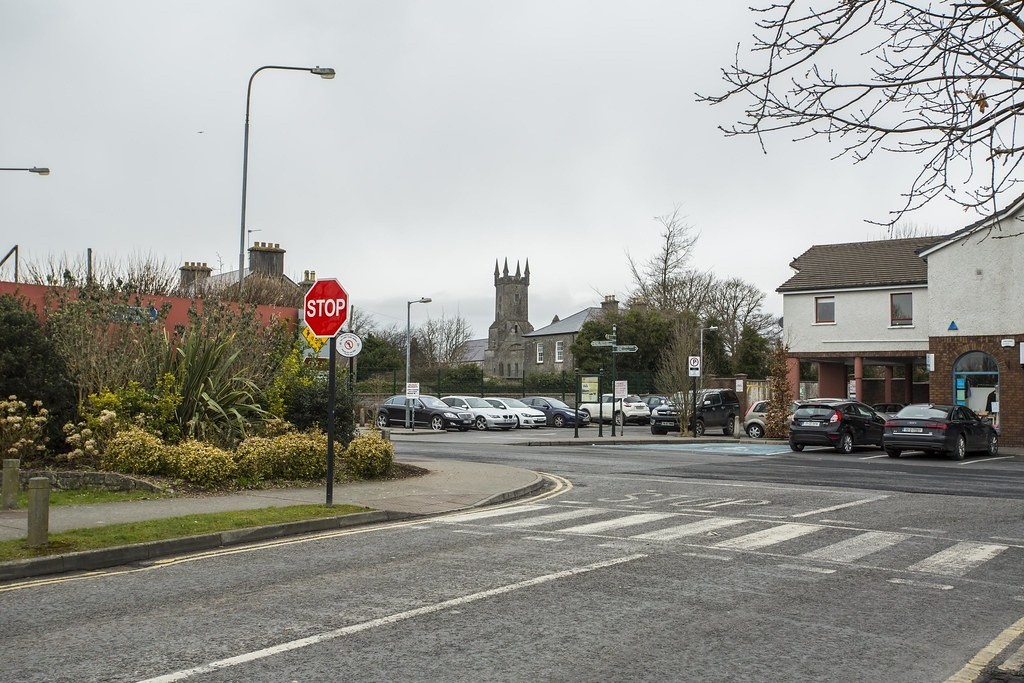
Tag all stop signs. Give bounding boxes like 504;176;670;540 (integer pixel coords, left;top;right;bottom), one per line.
305;277;349;339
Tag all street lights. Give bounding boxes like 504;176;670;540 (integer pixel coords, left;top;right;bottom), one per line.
698;324;719;391
404;295;433;429
238;63;335;297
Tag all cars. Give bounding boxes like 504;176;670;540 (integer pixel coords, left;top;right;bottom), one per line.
882;401;999;461
486;397;547;428
440;397;518;432
871;403;908;419
372;394;476;433
519;397;592;427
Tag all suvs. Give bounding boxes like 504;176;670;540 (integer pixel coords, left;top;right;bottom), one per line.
742;396;871;439
579;393;650;427
650;389;739;437
789;399;889;454
639;393;667;415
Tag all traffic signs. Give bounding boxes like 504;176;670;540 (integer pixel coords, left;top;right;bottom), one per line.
612;344;638;352
591;340;613;346
605;334;615;340
